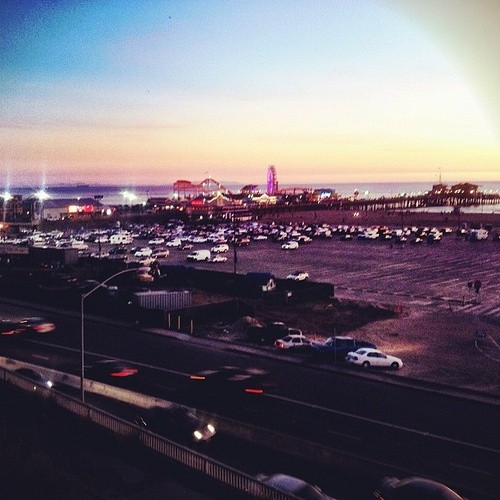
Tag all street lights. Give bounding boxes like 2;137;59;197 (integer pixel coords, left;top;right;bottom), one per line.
81;266;152;404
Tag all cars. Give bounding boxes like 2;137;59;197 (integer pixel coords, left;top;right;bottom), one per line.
267;322;404;371
88;359;141;380
3;224;490;264
372;478;462;500
285;270;309;283
0;319;24;339
18;316;58;335
242;473;333;500
